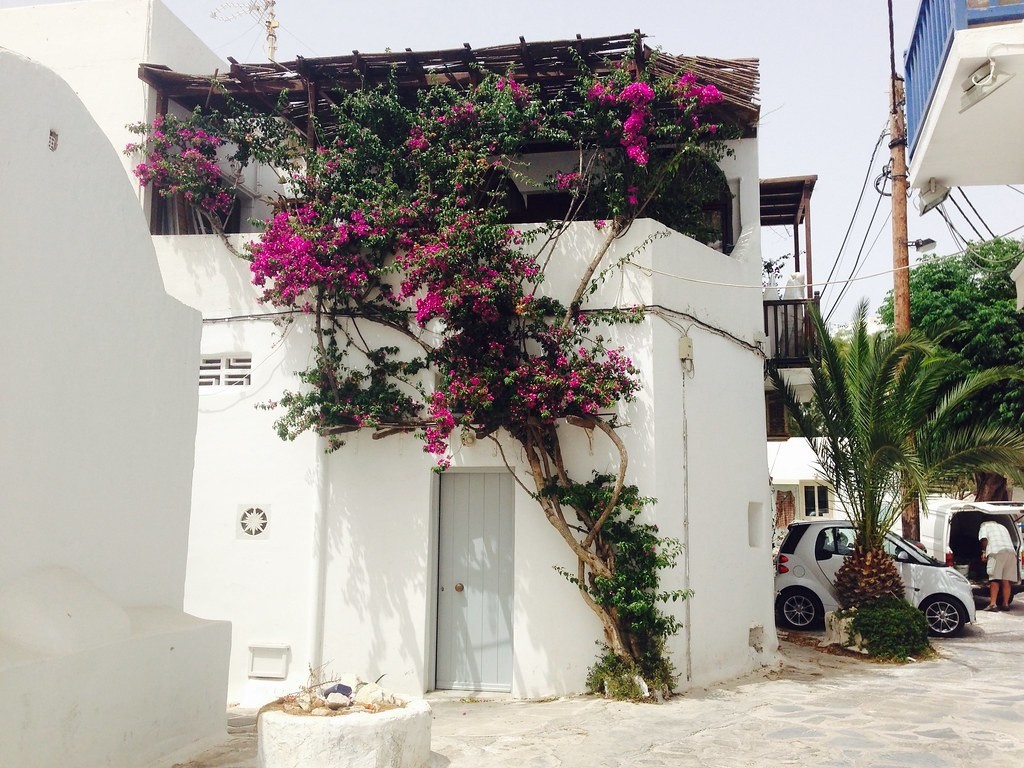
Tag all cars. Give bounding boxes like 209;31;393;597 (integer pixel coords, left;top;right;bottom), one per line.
773;519;977;637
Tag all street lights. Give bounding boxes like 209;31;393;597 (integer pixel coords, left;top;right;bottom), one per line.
891;72;936;541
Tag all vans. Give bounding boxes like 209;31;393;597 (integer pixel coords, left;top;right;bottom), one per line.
889;499;1024;607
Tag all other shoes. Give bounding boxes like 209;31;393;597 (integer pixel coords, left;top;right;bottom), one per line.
983;604;998;612
997;604;1010;611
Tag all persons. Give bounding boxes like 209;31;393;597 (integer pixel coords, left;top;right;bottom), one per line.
978;517;1018;612
